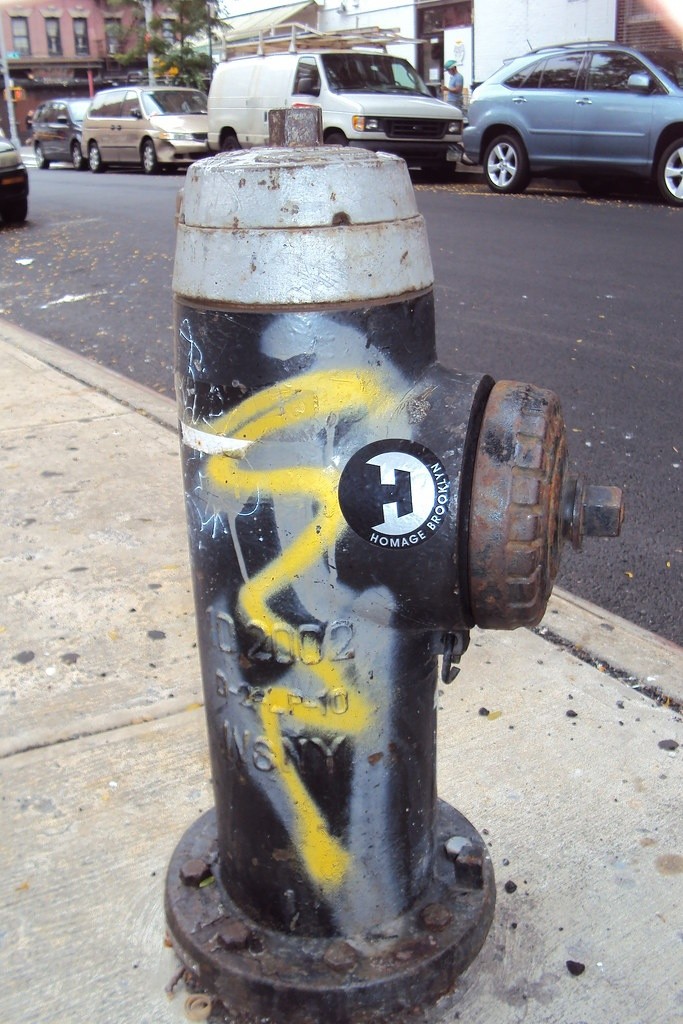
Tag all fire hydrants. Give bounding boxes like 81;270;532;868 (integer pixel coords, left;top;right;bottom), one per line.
162;102;626;1023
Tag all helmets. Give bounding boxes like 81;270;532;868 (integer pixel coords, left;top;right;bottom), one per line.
444;59;456;70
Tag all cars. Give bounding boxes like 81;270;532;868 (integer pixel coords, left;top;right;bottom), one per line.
0;128;29;225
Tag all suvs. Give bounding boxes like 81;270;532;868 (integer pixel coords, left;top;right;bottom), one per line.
460;41;683;206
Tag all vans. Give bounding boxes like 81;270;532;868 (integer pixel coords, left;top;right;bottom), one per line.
31;98;94;171
206;50;469;181
81;86;208;176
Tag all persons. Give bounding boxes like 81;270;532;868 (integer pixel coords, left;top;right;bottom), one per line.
441;60;464;107
24;109;34;145
